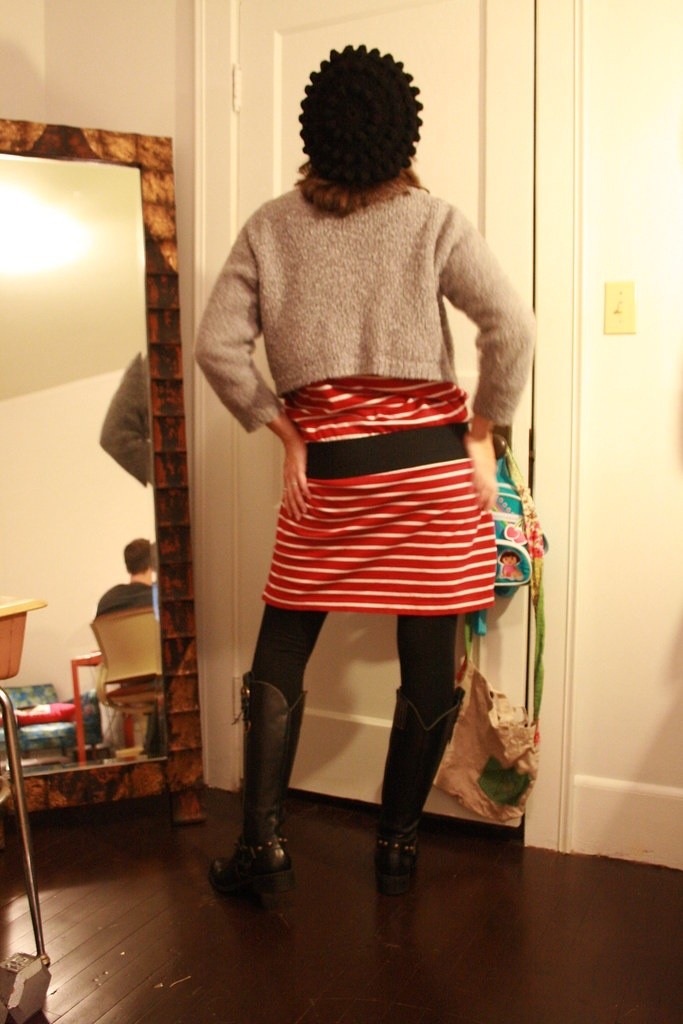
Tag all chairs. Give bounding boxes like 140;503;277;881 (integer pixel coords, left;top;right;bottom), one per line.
0;599;51;968
92;607;164;763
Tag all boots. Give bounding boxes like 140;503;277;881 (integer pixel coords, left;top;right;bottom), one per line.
203;675;307;887
372;685;466;894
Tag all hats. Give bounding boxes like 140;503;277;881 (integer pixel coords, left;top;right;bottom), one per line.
298;44;423;186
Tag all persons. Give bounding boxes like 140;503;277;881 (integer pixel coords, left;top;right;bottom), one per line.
195;45;536;894
91;538;165;758
100;352;152;488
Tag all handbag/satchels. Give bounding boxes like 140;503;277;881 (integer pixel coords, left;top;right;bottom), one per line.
432;666;541;820
455;458;532;636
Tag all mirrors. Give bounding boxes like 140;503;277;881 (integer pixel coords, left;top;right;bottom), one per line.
0;116;232;825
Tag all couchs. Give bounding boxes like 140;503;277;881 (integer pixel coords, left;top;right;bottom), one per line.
0;682;105;762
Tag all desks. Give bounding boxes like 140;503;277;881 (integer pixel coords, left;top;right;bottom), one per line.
71;649;137;767
